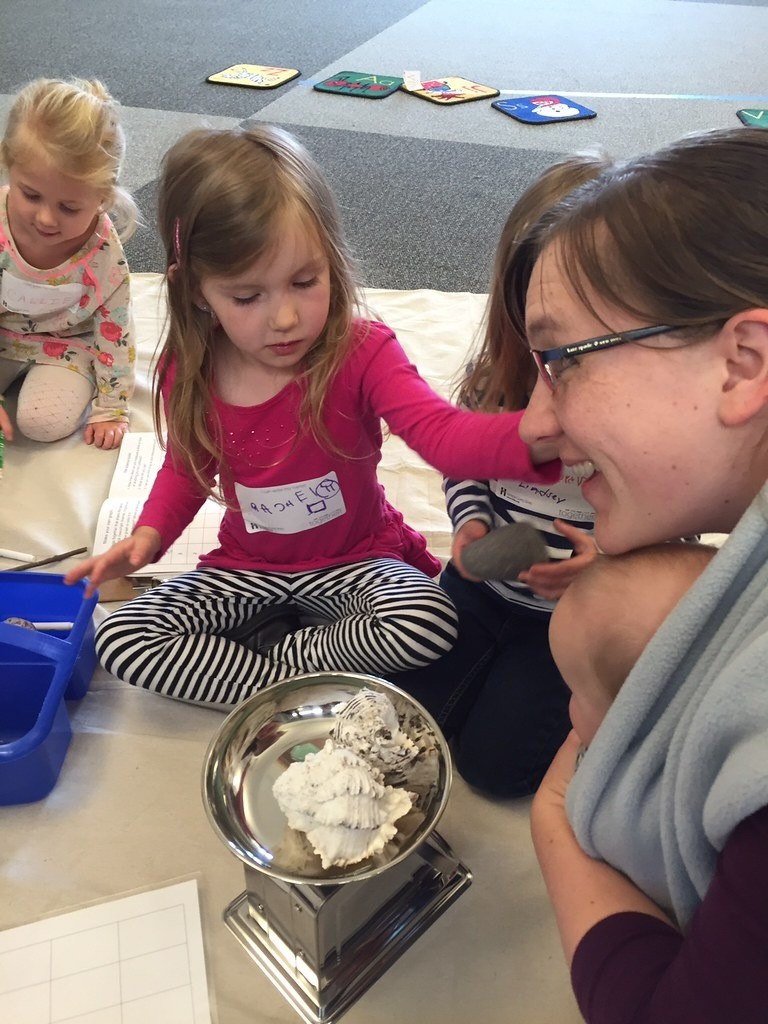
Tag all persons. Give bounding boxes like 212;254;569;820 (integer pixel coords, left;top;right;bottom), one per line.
385;151;703;799
501;126;768;1024
62;118;563;707
0;77;142;450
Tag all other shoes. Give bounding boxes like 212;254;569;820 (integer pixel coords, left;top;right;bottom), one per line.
218;603;303;654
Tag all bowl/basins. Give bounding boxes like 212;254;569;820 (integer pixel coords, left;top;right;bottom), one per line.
203;671;453;885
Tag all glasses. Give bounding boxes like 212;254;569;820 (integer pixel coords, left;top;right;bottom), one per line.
526;318;681;398
